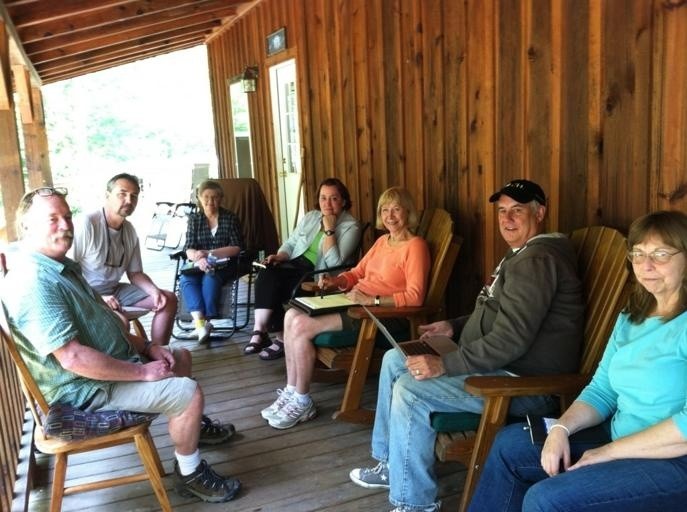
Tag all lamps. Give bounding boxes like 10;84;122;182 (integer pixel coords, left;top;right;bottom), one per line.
242;63;258;94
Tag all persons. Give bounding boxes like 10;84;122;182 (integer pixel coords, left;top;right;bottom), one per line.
244;180;360;359
259;188;430;430
179;182;241;343
66;174;177;348
350;180;586;512
2;188;240;503
467;211;686;511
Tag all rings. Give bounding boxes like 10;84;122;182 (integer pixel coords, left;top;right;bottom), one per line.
415;369;420;375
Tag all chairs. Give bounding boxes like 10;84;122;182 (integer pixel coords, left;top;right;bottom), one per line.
393;225;631;512
314;208;462;423
116;308;151;341
168;178;260;341
0;324;174;512
289;219;371;299
144;163;211;252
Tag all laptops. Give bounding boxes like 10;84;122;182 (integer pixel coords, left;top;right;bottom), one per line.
361;304;459;365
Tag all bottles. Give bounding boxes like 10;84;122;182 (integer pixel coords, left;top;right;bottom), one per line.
206;247;215;276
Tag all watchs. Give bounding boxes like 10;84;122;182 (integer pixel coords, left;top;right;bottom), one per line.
323;231;335;235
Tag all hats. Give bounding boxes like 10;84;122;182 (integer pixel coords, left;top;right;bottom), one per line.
489;178;546;207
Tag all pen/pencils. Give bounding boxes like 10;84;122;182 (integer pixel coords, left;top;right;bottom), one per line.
321;273;326;298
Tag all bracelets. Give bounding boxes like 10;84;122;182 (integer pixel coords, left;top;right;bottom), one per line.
547;424;572;436
375;294;381;306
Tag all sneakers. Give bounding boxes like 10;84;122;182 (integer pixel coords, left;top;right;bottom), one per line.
347;460;394;491
196;319;213;344
197;413;237;447
257;384;293;422
186;329;197;340
172;456;242;505
267;394;320;429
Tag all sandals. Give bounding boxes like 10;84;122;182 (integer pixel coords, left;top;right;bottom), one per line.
243;329;273;355
258;339;285;360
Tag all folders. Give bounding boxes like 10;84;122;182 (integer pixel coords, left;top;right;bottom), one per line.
288;293;361;317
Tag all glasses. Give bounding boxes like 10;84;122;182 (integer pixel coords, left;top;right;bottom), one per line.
21;186;68;213
625;245;683;265
